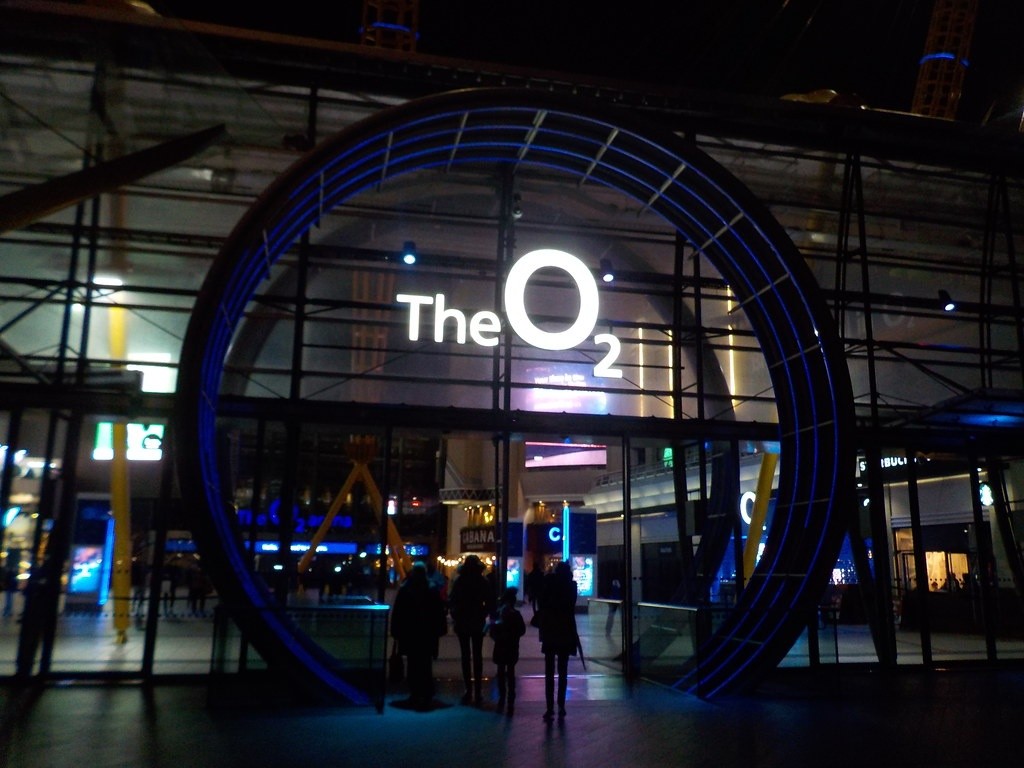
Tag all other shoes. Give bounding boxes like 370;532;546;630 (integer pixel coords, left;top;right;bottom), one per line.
496;706;504;712
506;706;515;719
463;693;473;701
544;713;554;721
558;709;566;720
474;697;490;705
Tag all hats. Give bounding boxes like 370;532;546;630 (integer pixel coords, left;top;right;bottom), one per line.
503;588;517;604
457;555;486;574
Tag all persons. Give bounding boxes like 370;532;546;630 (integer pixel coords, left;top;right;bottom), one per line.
604;578;621;639
530;561;577;718
892;574;968;625
2;553;67;676
446;555;492;703
488;588;526;707
113;553;214;619
401;559;554;635
388;565;448;711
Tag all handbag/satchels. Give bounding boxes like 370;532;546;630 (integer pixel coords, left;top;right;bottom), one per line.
387;641;407;696
